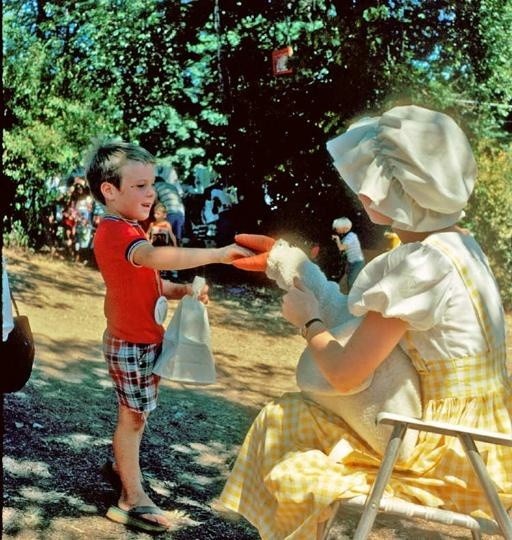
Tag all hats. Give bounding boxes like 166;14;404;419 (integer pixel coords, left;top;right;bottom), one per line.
325;105;478;232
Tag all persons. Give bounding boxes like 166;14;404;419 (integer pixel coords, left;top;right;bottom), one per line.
331;216;367;293
145;203;179;279
86;139;258;535
150;174;188;281
2;240;15;392
213;105;511;539
48;174;106;267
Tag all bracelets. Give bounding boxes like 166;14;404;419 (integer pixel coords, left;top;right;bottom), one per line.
301;317;323;338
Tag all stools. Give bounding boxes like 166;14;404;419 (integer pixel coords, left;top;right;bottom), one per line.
317;409;512;539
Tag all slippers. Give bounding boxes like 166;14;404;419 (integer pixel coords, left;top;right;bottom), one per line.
104;461;155;499
107;504;167;535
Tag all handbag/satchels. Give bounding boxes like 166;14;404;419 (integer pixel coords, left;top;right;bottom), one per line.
1;314;35;394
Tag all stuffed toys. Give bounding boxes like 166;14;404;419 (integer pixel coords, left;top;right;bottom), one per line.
230;232;423;460
